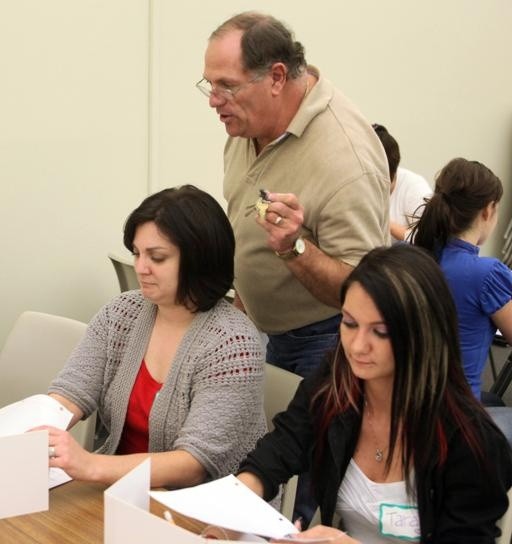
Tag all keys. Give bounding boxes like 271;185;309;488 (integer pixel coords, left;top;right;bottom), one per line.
244;190;271;220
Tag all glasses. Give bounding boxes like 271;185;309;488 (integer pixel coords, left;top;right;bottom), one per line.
196;72;269;101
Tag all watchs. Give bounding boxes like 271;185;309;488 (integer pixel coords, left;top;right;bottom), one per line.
274;234;306;260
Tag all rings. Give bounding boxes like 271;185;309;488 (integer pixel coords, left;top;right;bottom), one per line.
49;447;54;458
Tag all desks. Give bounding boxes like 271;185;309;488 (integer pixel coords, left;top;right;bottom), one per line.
1;479;287;544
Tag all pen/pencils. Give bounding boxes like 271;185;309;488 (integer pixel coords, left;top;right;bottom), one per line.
164;510;176;525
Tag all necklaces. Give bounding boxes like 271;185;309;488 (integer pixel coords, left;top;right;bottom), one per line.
361;392;400;461
303;75;311;100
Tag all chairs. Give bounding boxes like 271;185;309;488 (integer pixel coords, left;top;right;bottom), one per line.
257;359;308;523
1;311;96;451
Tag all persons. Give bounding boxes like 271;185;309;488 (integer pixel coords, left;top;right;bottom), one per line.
24;184;283;538
372;124;511;408
202;10;391;378
201;244;512;544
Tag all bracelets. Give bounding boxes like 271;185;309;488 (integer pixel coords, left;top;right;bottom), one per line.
201;524;229;541
